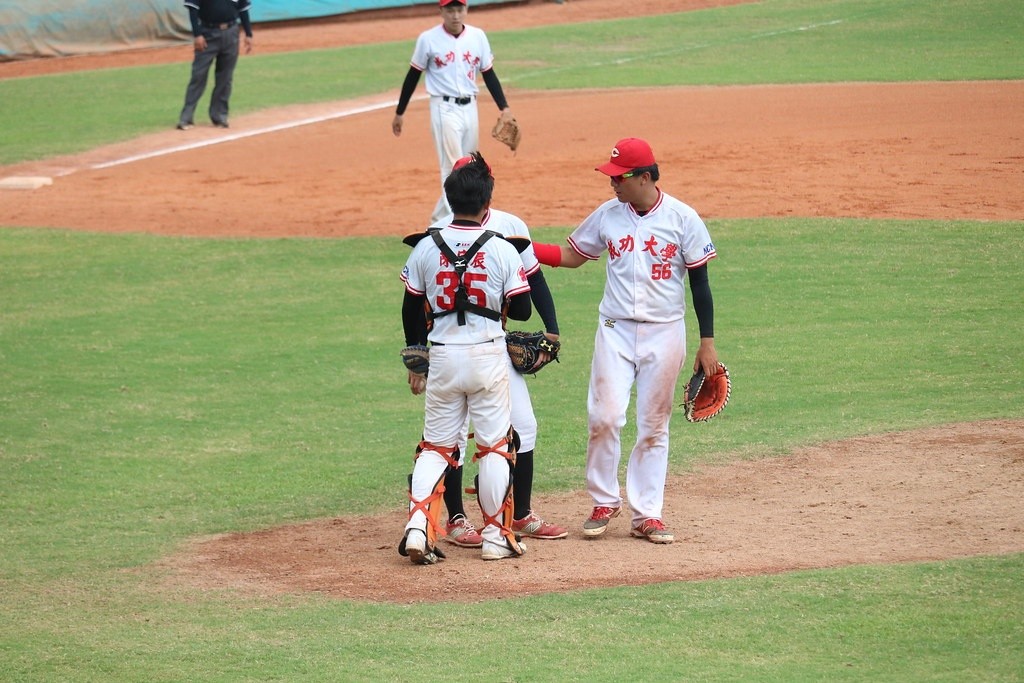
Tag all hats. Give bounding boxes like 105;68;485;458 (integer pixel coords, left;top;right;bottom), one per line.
452;155;495;181
439;0;466;8
595;137;657;176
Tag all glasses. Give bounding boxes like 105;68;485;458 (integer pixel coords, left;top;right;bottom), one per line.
610;173;635;184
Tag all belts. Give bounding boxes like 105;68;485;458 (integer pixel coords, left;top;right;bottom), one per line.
430;339;494;346
443;95;470;104
200;21;237;30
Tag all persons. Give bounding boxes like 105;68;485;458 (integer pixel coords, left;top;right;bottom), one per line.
175;0;253;130
392;0;521;226
533;137;719;544
396;151;570;565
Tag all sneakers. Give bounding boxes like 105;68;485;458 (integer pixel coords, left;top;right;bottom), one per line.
583;506;622;537
630;519;674;544
511;509;568;539
481;539;526;560
405;528;438;563
443;513;482;547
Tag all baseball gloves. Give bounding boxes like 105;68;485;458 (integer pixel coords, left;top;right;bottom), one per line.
684;361;732;422
490;113;523;151
507;330;561;376
400;344;431;377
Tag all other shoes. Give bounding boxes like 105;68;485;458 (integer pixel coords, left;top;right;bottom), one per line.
214;118;230;129
177;120;193;129
